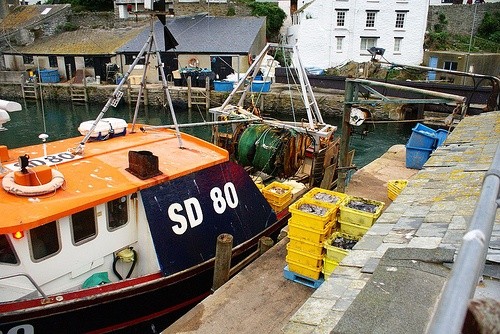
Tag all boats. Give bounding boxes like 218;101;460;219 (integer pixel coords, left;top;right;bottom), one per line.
0;8;360;334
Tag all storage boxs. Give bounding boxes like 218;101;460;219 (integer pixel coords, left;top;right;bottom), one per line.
40;70;60;83
285;187;385;280
387;180;408;201
405;123;452;169
129;75;143;85
250;80;272;93
213;80;235;92
262;181;293;213
